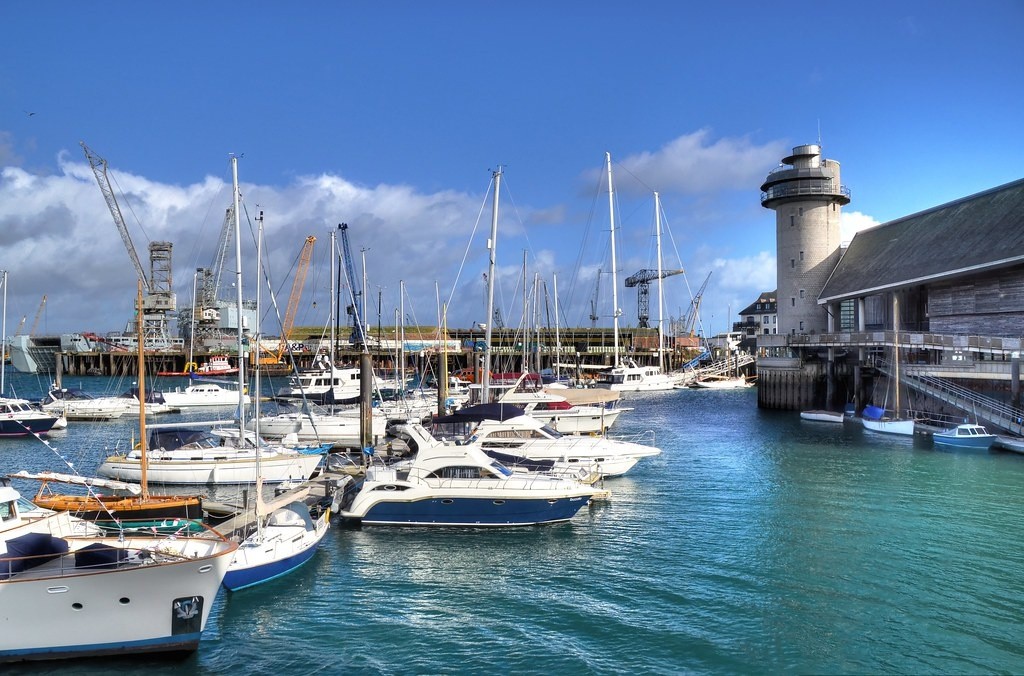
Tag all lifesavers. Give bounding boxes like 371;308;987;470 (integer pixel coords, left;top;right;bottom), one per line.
387;360;392;373
593;373;598;378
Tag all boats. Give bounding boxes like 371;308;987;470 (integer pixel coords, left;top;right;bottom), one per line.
0;475;241;662
331;416;611;525
40;380;177;416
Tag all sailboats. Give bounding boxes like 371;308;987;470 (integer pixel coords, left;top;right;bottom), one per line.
161;270;255;407
0;270;58;435
217;204;331;590
96;150;754;483
